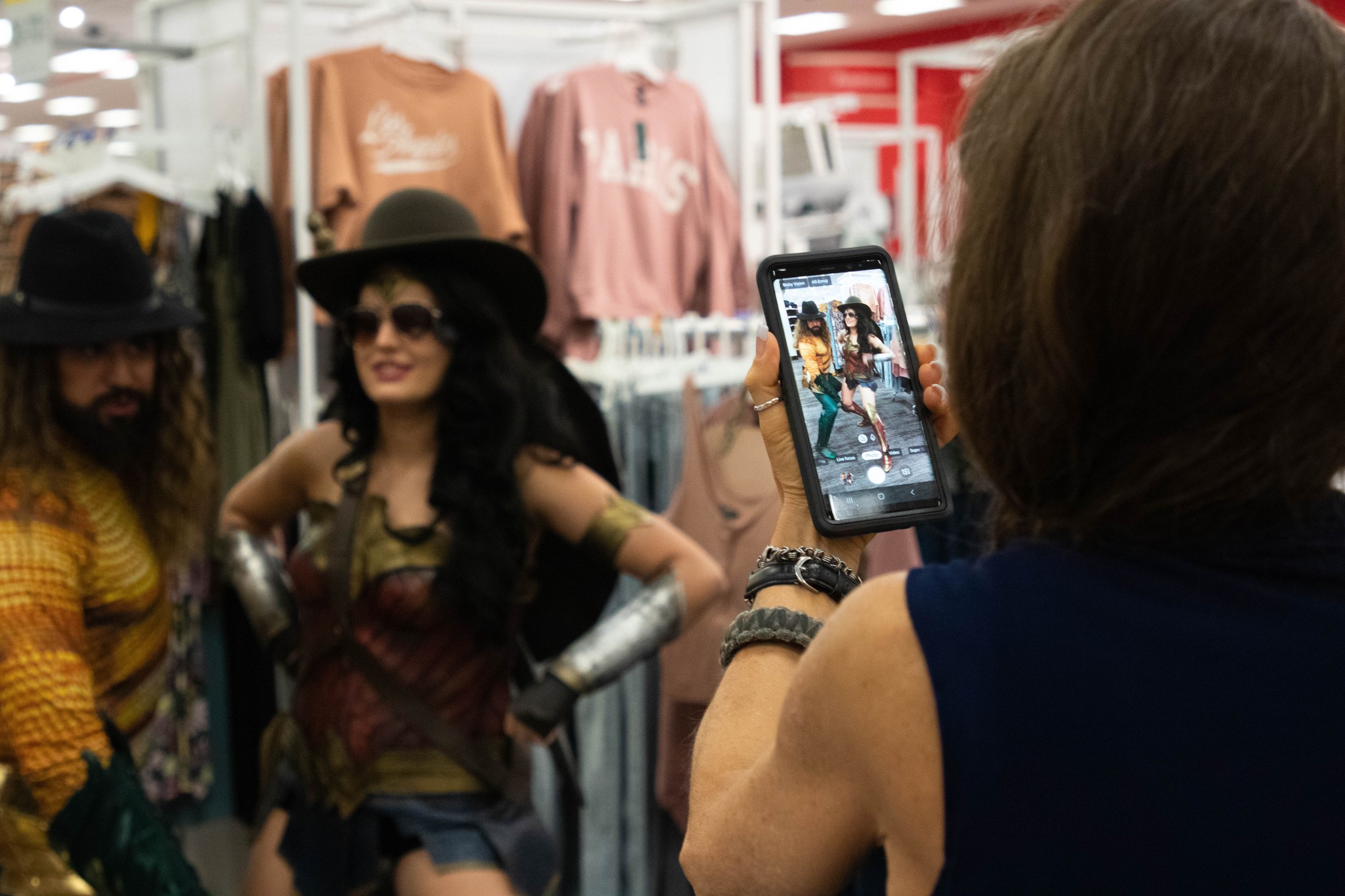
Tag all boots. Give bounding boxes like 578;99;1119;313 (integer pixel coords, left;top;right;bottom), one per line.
815;413;836;460
873;420;892;472
840;400;872;426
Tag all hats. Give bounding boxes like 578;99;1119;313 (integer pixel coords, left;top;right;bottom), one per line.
837;296;872;317
795;301;826;319
295;189;549;330
1;206;199;352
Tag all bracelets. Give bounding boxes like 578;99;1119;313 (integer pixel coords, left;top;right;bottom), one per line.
720;606;824;674
756;545;863;586
744;557;859;609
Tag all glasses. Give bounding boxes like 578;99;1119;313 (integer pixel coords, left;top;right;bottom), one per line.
340;303;433;340
842;312;854;319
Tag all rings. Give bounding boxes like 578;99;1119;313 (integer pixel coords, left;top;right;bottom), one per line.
753;395;782;411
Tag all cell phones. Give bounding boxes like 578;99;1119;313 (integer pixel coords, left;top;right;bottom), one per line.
755;244;954;536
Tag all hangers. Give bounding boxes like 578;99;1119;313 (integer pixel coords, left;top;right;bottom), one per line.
0;119;273;225
268;3;707;108
565;311;766;417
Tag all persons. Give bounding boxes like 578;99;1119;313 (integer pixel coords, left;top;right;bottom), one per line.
220;184;730;896
0;202;214;896
679;0;1345;896
793;297;896;473
